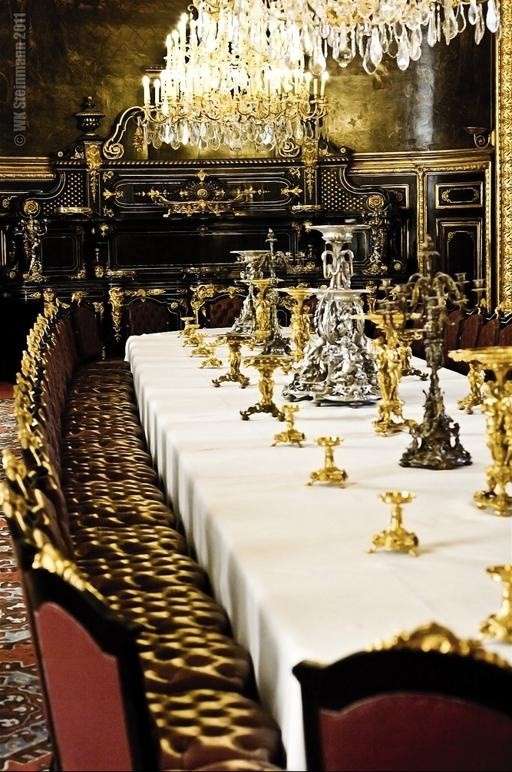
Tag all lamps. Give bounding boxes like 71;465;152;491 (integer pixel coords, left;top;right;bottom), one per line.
137;0;501;150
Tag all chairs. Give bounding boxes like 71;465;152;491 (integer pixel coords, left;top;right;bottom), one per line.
0;282;512;772
